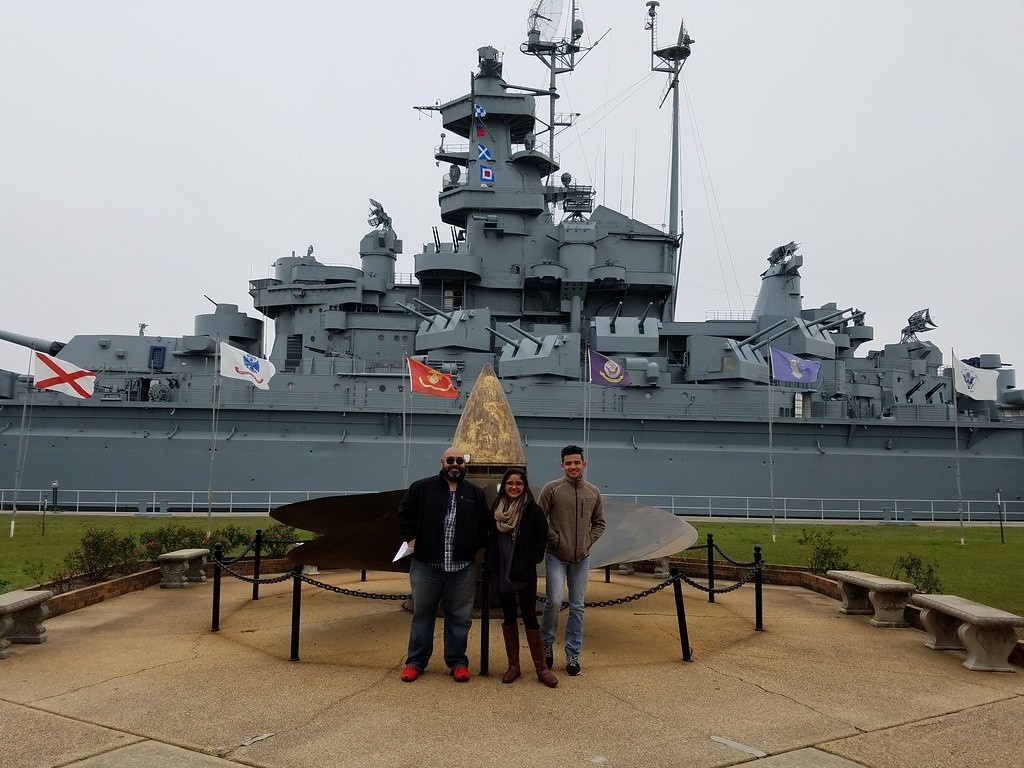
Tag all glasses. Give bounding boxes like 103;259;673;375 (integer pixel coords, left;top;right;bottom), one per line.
447;459;464;465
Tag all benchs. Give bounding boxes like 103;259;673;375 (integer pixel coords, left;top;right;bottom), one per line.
0;591;54;659
912;594;1024;673
156;549;210;589
827;570;916;628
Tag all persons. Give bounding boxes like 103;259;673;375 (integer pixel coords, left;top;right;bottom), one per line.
401;447;490;683
421;357;427;365
485;468;558;687
537;445;607;676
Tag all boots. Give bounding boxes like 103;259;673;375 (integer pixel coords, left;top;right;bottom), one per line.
525;625;557;687
502;623;521;683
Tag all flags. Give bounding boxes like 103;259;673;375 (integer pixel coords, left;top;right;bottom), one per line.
477;143;491;160
33;351;98;399
474;103;486;117
590;350;631;387
953;354;1001;400
479;164;494;182
406;357;457;399
219;341;276;390
770;346;822;383
476;125;486;136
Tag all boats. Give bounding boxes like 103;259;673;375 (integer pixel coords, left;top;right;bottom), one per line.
0;1;1024;525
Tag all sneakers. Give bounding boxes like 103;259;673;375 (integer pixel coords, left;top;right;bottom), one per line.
451;667;470;682
544;646;554;669
566;655;581;676
402;665;420;682
